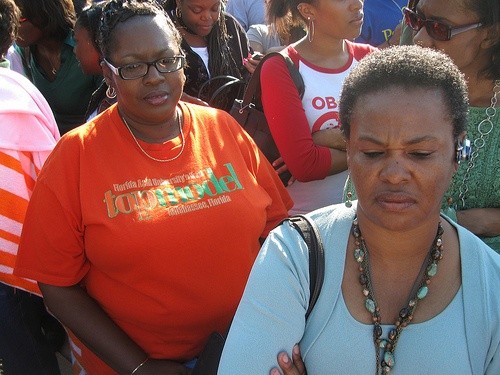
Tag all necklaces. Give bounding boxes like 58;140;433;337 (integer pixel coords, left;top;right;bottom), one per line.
41;43;61;75
457;80;500;208
122;106;185;162
352;218;445;375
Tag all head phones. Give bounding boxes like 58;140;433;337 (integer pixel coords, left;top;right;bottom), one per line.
456;138;471;164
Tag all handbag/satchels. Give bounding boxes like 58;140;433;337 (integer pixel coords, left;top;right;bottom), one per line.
191;332;225;375
230;52;305;188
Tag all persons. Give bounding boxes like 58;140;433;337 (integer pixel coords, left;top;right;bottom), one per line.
162;1;266;113
352;0;409;50
8;0;93;138
224;0;308;56
342;0;500;253
73;6;118;126
0;0;22;69
260;0;381;219
12;0;295;375
0;66;61;375
217;45;500;375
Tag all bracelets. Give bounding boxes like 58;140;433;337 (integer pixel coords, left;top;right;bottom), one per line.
131;358;149;375
387;39;391;46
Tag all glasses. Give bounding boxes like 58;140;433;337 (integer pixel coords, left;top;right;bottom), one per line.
402;8;484;42
103;48;186;81
